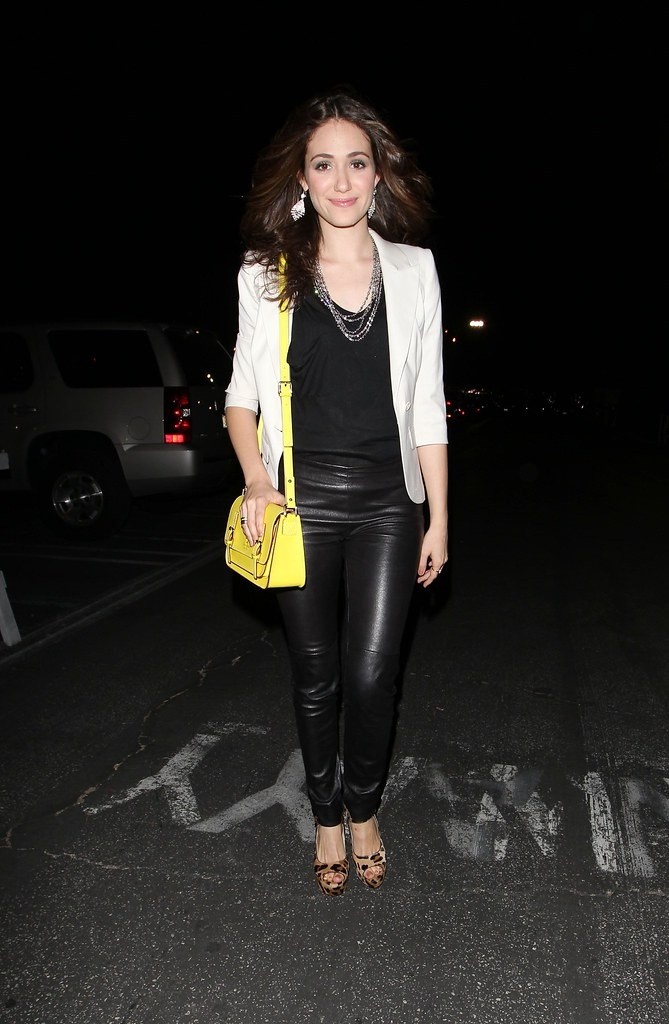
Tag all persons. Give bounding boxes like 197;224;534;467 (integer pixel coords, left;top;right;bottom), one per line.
222;91;450;900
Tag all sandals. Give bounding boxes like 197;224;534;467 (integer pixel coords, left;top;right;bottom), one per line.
348;815;387;888
314;824;350;895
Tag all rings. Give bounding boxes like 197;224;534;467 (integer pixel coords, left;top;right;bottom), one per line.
431;566;441;574
240;519;247;525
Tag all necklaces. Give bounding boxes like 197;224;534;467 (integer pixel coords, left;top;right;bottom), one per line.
313;235;383;344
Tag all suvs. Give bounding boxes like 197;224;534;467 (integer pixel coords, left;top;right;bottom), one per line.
0;322;240;543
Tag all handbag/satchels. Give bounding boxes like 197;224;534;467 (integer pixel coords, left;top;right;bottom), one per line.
223;495;306;592
